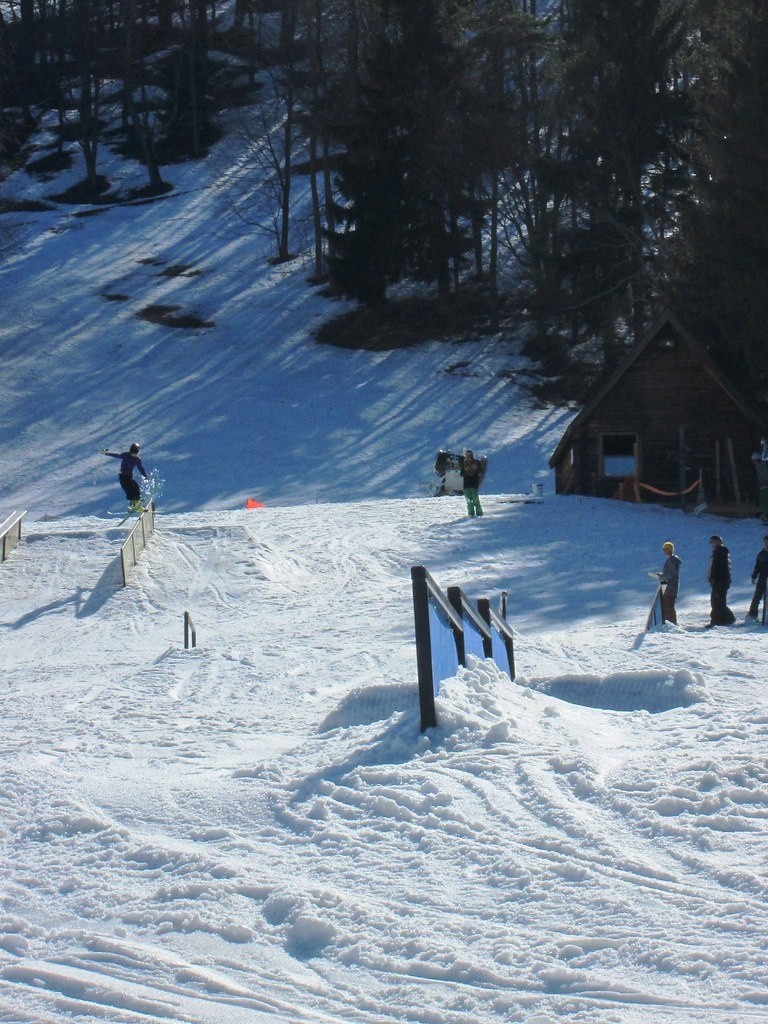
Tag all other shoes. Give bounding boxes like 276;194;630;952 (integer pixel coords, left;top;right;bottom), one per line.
129;500;148;512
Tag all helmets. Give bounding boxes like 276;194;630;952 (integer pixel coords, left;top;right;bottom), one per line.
132;443;140;451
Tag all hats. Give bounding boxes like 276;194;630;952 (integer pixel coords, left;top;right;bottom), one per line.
663;542;673;550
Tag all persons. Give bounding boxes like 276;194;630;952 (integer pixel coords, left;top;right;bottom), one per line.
658;542;682;622
101;442;149;509
458;450;484;518
747;537;768;624
752;453;768;525
704;535;736;629
433;449;452;495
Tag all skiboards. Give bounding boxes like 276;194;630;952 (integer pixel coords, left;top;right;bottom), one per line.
108;487;164;527
